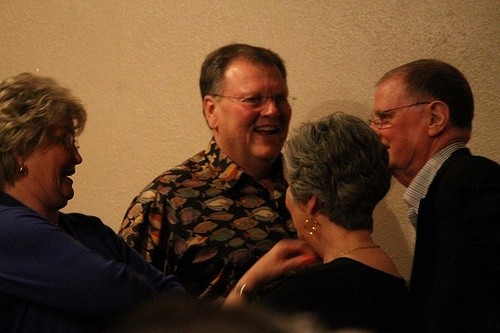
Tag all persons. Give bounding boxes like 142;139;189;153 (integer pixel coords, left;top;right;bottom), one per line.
222;112;428;333
118;45;313;308
366;59;500;333
0;73;197;333
166;306;332;333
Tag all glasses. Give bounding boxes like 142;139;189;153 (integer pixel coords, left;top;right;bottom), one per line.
43;133;80;151
368;102;429;128
207;93;297;107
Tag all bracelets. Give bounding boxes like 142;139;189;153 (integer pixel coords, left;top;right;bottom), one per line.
232;280;251;300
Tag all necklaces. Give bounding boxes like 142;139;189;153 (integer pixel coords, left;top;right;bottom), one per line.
331;243;383;258
